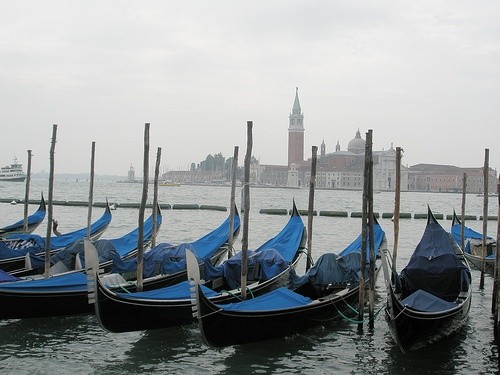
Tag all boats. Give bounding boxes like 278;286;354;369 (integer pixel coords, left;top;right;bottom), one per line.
0;202;166;283
0;162;29;184
0;192;47;235
160;183;181;187
195;212;388;346
451;209;496;267
0;204;241;314
387;203;472;351
0;198;112;271
95;196;308;333
